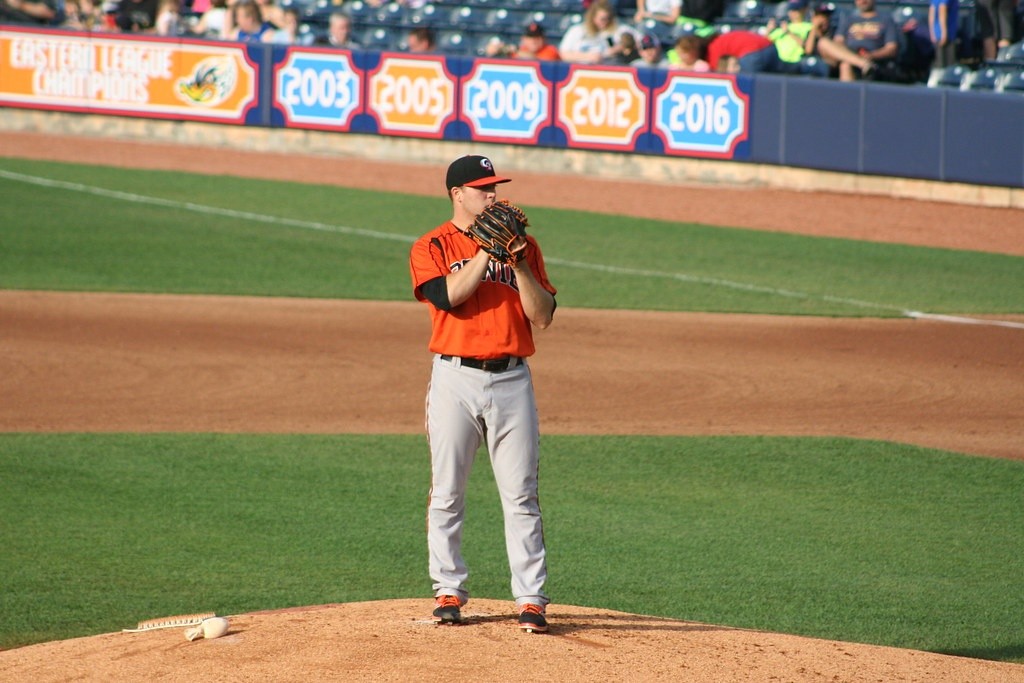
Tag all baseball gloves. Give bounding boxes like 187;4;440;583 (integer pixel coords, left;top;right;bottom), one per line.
461;197;531;269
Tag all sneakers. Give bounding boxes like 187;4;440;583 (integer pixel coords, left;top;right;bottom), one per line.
519;603;548;632
433;594;460;625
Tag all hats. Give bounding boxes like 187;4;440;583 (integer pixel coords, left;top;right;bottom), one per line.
637;33;660;49
524;20;544;37
675;23;698;38
813;4;834;15
446;154;512;190
788;0;804;9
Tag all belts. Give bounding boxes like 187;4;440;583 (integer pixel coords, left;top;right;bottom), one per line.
441;355;524;373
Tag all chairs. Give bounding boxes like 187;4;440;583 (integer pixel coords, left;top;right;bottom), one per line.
186;0;1024;91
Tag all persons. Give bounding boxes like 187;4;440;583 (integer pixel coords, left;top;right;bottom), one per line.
407;153;559;633
0;0;1016;81
